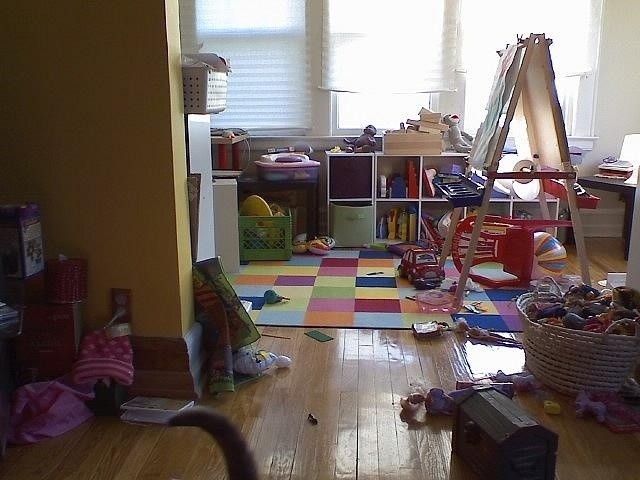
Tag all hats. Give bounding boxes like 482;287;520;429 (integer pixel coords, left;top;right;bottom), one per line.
73;329;135;386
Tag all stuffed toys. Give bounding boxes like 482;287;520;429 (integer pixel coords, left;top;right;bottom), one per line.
532;280;640;336
575;390;608;423
440;114;474;154
342;124;378;154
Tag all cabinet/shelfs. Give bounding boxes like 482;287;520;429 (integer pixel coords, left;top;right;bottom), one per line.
328;150;560;257
238;178;321;246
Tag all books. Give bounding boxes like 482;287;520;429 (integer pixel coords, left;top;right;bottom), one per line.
0;302;19;330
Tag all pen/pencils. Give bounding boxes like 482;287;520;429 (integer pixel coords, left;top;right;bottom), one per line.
366;271;384;275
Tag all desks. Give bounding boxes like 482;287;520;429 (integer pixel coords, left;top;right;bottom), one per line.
563;176;638;261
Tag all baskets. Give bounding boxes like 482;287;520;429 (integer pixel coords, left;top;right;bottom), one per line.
238;206;293;261
515;276;640;397
46;257;88;303
181;68;228;114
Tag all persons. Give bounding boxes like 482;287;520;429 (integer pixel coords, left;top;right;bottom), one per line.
454;317;523;350
398;382;515;415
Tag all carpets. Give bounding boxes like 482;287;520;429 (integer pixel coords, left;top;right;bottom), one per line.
229;247;526;333
